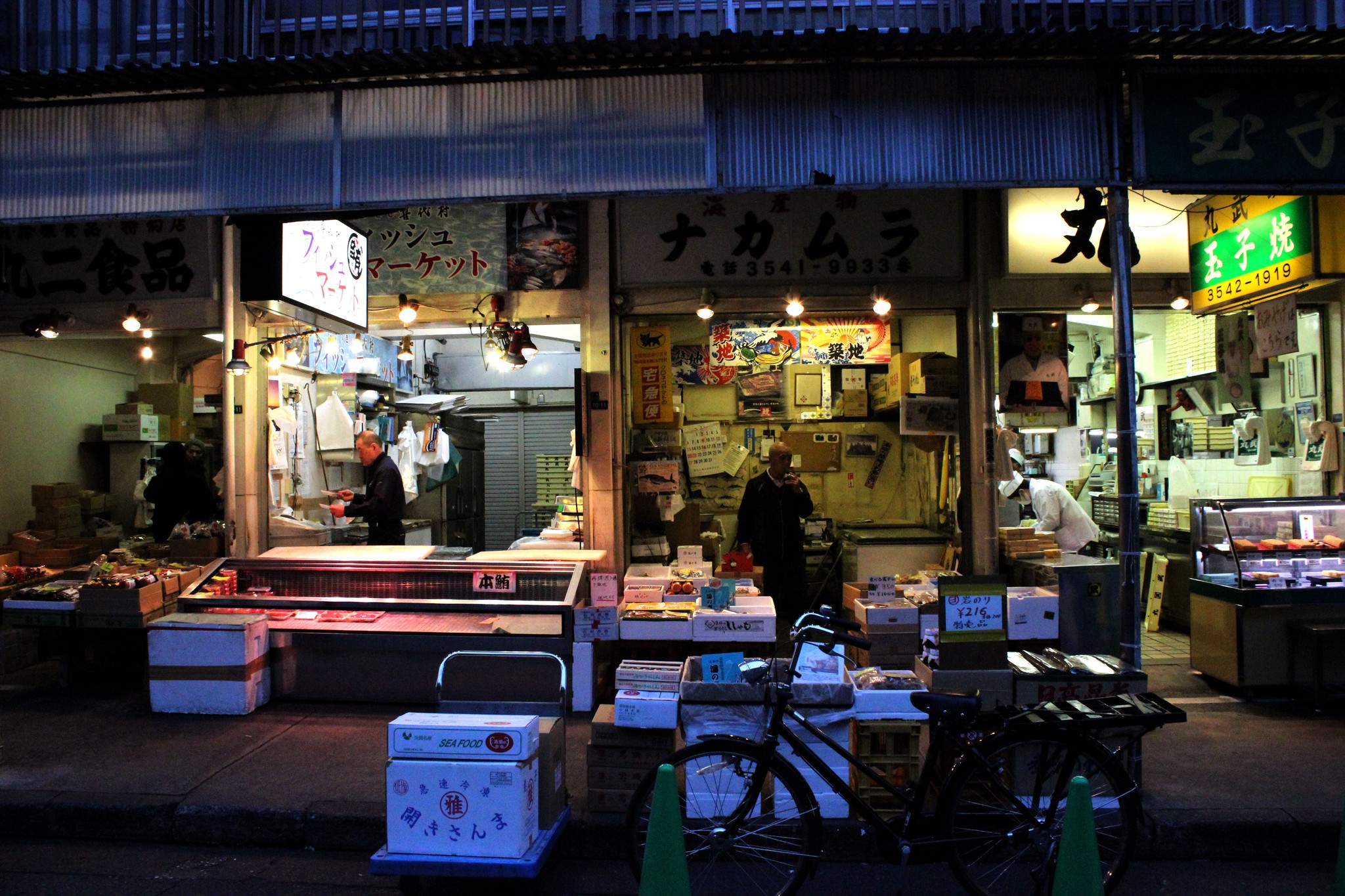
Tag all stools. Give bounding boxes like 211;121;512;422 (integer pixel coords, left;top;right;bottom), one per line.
1286;621;1345;716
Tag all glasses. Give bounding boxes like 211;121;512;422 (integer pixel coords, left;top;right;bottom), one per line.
357;445;370;454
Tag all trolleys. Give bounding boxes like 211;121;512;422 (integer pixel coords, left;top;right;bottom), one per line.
371;650;575;895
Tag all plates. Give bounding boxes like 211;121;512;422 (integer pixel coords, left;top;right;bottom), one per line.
321;490;341;496
319;504;330;508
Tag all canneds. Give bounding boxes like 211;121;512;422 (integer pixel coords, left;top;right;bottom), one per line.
666;581;699;595
81;574;158;589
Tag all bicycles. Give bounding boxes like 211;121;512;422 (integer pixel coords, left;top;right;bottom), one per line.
626;610;1189;896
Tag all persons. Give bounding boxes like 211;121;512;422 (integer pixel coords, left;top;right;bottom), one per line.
1223;321;1243;389
329;430;405;545
999;318;1067;413
144;440;212;546
999;470;1099;556
737;441;814;606
1009;448;1024;471
185;441;205;464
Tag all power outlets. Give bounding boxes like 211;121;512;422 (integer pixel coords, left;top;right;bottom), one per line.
282;382;301;399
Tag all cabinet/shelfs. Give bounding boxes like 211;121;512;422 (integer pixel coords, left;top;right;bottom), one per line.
315;373;395;463
1189;496;1345;688
1024;454;1053;480
1092;496;1223;632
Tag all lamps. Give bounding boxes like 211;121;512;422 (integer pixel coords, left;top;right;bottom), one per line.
785;286;804;317
1165;279;1189;310
1074;279;1101;314
873;282;891;315
696;288;714;319
486;295;539;369
1017;425;1059;432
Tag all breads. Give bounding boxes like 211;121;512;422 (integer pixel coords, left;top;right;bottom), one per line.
998;526;1062;559
1233;534;1345;550
1252;571;1279;580
1322;570;1345;578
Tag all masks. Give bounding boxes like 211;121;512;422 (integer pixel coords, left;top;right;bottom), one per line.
1017;493;1031;505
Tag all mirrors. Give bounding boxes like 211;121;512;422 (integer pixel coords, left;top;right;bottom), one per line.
1170;307;1327;459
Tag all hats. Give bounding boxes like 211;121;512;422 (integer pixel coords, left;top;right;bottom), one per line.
1010;452;1024;467
1009;448;1021;455
184;439;205;457
998;470;1024;497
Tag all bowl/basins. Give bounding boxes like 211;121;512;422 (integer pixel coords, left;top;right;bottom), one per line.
700;514;714;521
203;583;222;595
210;608;386;621
219;567;237;597
213;576;230;596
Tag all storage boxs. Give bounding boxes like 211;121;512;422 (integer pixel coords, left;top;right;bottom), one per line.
387;383;1013;860
840;352;958;418
348;357;381;377
1181;417;1235;450
357;389;401;441
1065;462;1239;631
0;384;272;716
421;421;440;453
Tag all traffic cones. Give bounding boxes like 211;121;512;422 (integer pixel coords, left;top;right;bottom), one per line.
1052;774;1108;896
638;764;693;896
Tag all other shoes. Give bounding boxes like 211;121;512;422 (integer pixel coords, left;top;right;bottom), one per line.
797;624;812;639
